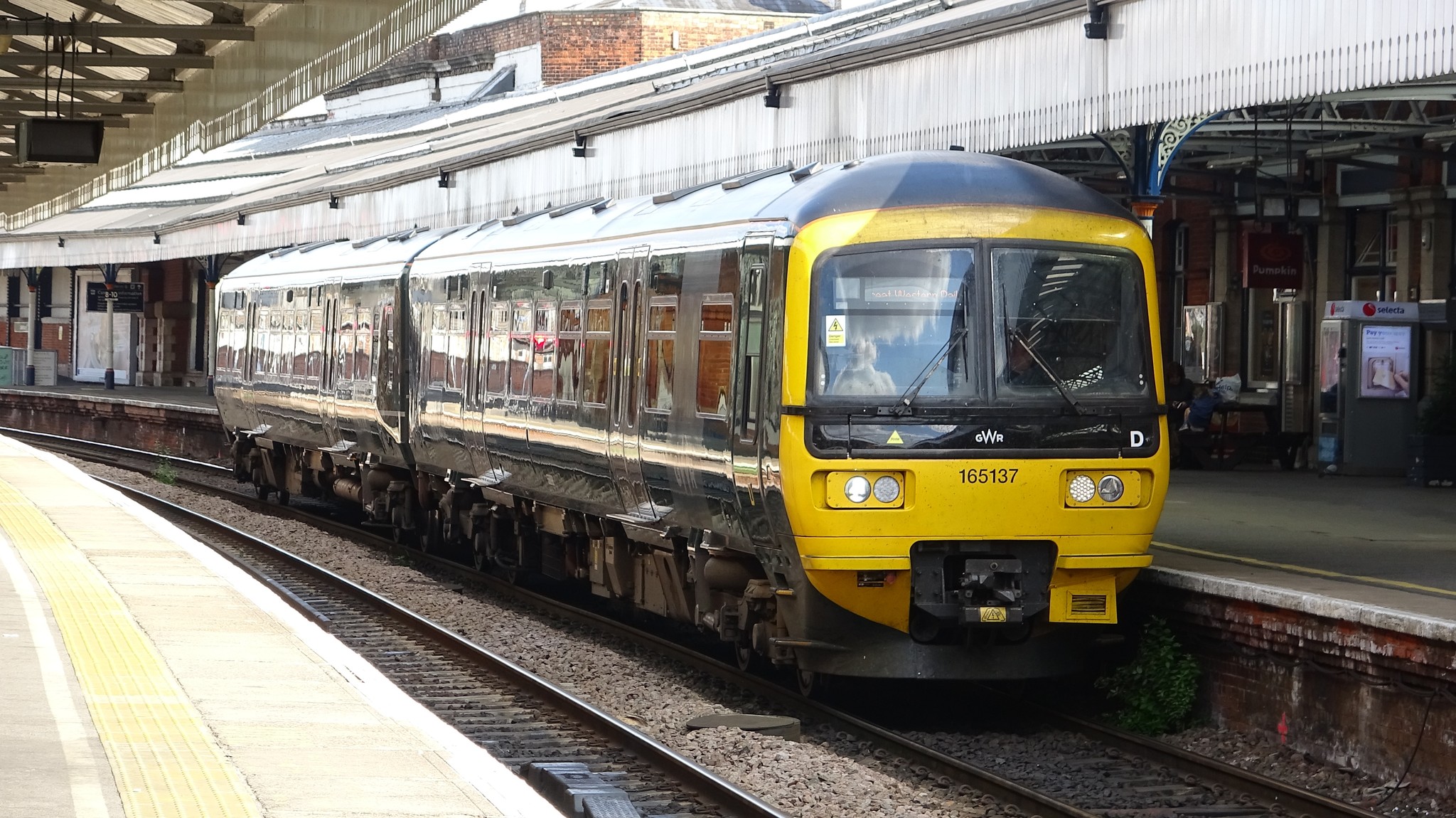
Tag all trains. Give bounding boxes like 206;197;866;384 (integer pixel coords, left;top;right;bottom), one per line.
213;149;1172;695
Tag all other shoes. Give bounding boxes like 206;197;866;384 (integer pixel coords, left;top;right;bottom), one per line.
1179;424;1189;431
1191;426;1205;432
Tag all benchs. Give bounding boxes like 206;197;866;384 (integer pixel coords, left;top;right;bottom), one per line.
1172;402;1300;472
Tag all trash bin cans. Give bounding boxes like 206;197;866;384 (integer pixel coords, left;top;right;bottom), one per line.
1317;413;1338;469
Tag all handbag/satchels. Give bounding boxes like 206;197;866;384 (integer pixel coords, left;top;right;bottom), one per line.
1214;373;1241;403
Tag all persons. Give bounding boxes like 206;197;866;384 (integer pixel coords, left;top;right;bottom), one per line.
1393;369;1409;394
1178;385;1220;431
1006;331;1061;388
1163;361;1195;470
1186;324;1204;382
